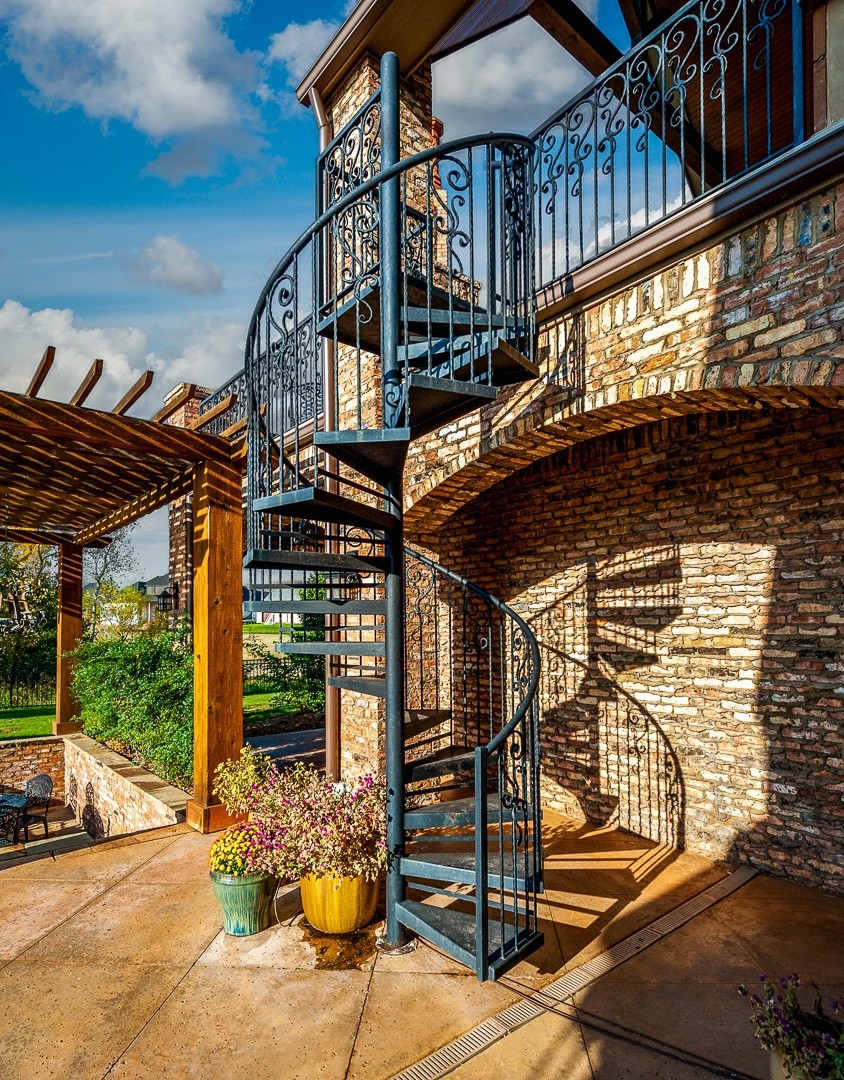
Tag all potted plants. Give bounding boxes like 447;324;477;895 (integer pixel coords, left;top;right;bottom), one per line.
208;744;386;935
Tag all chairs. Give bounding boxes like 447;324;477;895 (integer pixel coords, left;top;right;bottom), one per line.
5;773;54;842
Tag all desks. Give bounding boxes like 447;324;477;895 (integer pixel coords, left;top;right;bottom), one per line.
0;792;27;842
